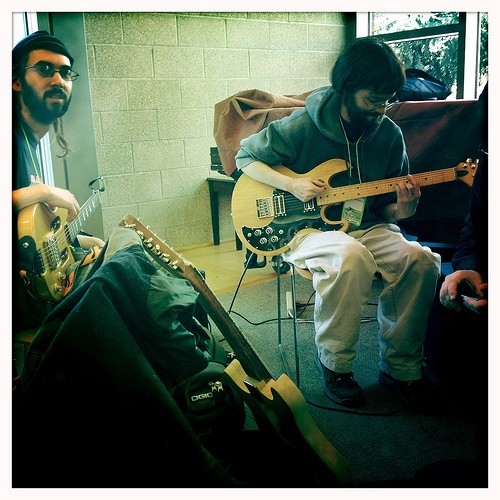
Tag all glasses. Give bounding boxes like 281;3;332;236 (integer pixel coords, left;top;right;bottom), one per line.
14;60;79;81
354;87;399;113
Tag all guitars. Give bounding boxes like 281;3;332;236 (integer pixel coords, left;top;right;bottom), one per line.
117;214;345;482
230;157;482;257
17;189;103;305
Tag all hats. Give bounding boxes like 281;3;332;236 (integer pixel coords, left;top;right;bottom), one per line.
12;30;74;65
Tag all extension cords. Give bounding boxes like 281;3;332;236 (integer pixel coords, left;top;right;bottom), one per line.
286;291;293;316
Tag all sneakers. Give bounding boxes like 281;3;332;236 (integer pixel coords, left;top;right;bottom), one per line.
314;348;363;405
379;371;434;393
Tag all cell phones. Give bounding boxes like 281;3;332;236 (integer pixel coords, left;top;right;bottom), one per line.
461;294;481;301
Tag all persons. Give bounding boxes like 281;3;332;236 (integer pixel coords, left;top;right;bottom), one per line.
231;37;446;412
12;29;109;328
422;116;487;419
